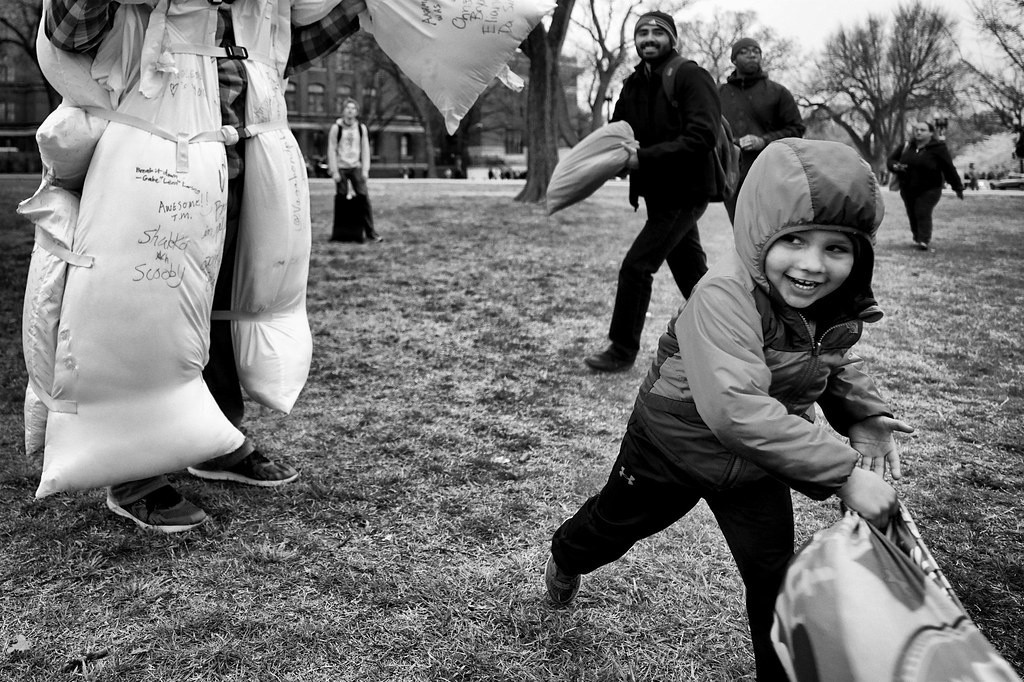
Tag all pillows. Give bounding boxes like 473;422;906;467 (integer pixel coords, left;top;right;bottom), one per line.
16;0;559;499
545;121;640;213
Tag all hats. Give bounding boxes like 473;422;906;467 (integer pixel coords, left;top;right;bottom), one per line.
731;39;761;62
635;10;677;46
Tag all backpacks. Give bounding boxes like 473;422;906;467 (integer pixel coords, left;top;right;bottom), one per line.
664;55;741;203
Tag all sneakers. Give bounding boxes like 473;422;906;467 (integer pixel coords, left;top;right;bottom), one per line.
544;517;581;605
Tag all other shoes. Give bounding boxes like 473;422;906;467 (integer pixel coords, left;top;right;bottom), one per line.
188;449;299;486
106;483;207;535
918;242;928;250
586;343;634;372
367;233;383;242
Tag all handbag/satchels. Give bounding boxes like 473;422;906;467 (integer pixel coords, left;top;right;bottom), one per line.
889;172;900;192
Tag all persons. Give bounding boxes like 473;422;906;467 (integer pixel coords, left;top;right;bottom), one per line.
581;12;723;371
15;0;370;536
886;121;964;250
545;136;915;682
968;163;979;191
716;37;807;227
327;97;385;243
